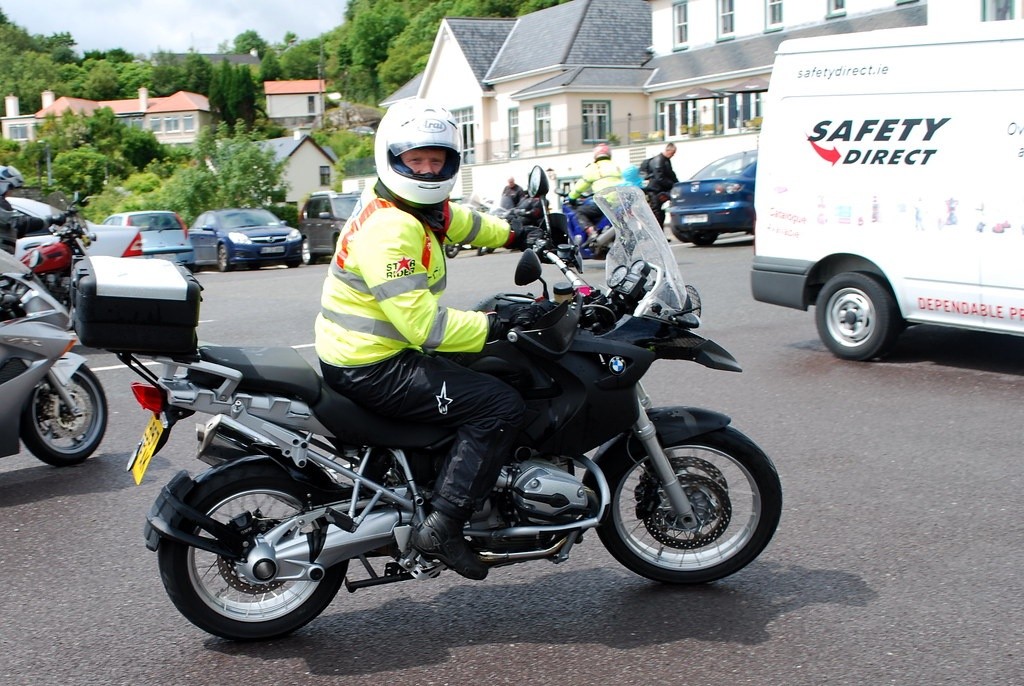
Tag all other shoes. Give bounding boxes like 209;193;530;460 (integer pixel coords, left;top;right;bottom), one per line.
411;515;488;581
581;230;598;249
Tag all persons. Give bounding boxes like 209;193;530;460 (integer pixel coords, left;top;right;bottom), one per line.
502;176;527;209
642;141;684;240
0;165;68;256
310;97;546;586
561;142;628;249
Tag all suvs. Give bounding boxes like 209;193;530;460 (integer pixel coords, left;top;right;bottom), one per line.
300;187;362;267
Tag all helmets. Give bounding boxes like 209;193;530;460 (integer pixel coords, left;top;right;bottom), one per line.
0;166;24;195
373;99;463;205
594;144;612;160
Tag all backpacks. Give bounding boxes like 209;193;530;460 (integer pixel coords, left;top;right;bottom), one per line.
639;154;670;196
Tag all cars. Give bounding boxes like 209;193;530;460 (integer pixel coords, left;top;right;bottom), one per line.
1;195;143;258
100;208;198;273
666;146;756;250
187;206;304;274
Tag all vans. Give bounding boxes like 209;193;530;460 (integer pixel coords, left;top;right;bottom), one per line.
753;13;1024;361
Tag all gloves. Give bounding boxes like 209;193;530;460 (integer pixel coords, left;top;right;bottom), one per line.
508;226;549;252
486;301;551;342
47;212;65;227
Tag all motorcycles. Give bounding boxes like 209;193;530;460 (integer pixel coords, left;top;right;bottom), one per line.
67;165;787;644
2;196;96;317
0;246;109;469
444;172;651;264
